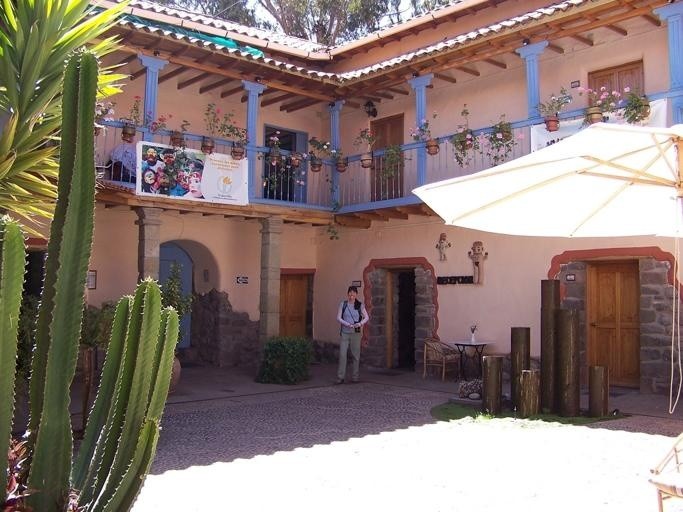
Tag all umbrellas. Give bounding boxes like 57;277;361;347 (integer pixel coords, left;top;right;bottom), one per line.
411;123;683;237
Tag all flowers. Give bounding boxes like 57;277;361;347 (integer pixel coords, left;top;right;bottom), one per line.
96;95;250;146
470;319;482;334
257;127;384;190
409;84;651;167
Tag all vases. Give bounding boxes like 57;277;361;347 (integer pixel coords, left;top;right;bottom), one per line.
468;333;476;343
264;187;279;200
201;140;243;160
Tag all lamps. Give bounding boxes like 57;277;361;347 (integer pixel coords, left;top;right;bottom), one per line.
362;99;377;119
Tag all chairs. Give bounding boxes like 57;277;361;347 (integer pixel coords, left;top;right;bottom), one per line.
421;337;465;383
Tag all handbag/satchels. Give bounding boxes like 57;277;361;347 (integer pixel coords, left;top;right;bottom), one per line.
354;321;361;332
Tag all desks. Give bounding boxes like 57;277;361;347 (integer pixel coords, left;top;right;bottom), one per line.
447;339;497;383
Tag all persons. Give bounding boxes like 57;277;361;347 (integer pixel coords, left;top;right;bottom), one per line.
142;145;205;199
335;286;369;385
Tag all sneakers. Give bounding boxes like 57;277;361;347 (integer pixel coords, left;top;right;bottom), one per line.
333;376;361;384
